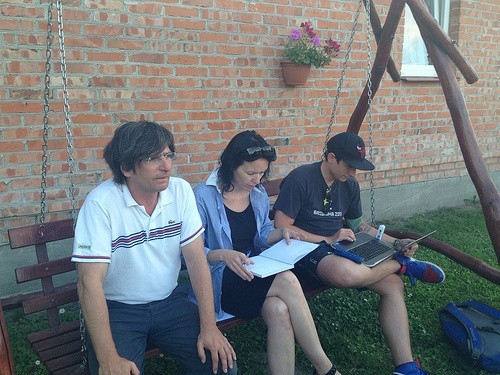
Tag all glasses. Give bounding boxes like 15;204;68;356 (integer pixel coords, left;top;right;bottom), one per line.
239;146;275;157
144;152;177;164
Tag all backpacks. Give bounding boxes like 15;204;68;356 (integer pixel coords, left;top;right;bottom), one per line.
438;301;500;375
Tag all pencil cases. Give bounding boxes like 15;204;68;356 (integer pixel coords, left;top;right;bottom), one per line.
335;247;363;264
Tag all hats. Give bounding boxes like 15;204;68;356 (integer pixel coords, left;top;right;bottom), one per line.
326;132;374;170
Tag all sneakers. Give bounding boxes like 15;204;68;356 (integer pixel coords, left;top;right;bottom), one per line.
393;254;445;283
393;362;427;375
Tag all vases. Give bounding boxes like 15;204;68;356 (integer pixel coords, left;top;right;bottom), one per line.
282;61;310;88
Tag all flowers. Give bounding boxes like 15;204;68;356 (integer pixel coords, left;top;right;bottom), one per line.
284;23;340;68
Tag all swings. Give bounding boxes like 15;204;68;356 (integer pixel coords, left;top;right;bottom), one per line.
7;0;376;375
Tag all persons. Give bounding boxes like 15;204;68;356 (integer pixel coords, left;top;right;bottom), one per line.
70;121;239;375
274;131;446;375
189;129;346;375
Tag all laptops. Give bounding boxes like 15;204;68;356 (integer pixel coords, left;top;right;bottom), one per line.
331;230;436;267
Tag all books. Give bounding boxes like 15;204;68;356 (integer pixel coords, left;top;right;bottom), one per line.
240;238;321;279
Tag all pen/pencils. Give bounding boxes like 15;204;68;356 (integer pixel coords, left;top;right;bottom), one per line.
241;250;251;265
